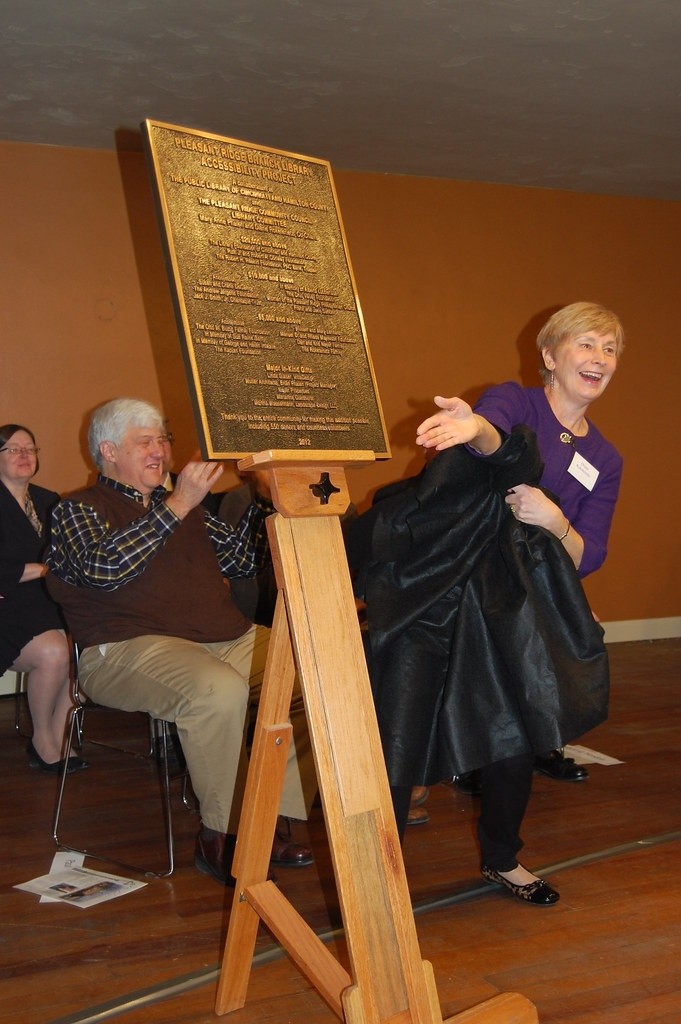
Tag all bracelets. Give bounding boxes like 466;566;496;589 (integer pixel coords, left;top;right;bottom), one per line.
559;519;570;540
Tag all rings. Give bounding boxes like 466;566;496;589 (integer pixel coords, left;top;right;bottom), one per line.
511;505;516;512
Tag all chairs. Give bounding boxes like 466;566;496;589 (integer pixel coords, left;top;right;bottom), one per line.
46;625;297;880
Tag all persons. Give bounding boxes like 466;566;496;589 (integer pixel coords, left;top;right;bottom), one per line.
43;399;318;888
391;302;623;906
218;482;430;824
0;424;90;774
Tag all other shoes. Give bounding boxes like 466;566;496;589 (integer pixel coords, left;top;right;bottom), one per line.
482;855;560;904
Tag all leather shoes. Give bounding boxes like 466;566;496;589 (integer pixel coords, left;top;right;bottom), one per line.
195;824;279;888
407;808;428;824
409;786;429;807
269;829;313;867
454;780;479;794
535;751;589;782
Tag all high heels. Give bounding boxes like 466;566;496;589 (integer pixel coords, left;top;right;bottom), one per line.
64;753;91;771
26;739;75;774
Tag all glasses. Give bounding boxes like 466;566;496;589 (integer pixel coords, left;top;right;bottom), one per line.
1;446;42;456
